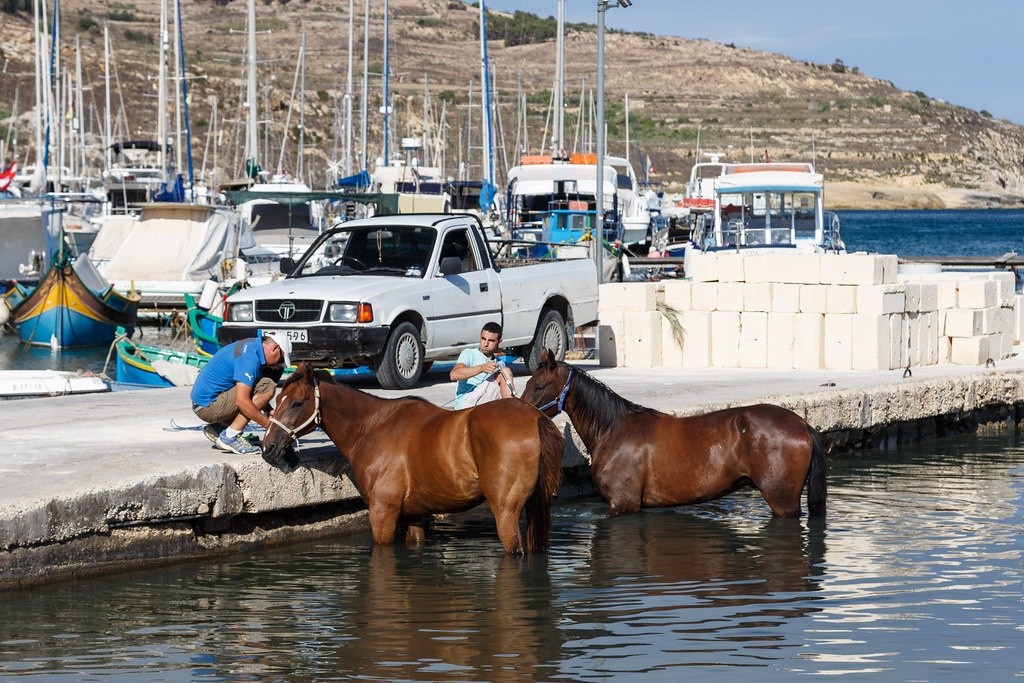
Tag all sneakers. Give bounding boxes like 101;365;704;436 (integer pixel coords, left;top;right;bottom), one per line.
216;430;261;454
203;421;226;442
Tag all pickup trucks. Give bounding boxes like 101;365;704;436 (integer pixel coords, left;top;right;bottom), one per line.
216;213;597;390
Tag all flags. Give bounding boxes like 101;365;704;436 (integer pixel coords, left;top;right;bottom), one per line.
0;160;17;191
646;154;656;183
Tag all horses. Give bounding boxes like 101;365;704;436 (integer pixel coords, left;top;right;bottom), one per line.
519;346;828;519
260;363;566;555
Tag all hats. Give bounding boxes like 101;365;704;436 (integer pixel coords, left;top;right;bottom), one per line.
265;330;293;368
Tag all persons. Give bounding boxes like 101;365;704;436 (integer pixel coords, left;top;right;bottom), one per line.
450;322;517;410
190;331;293;455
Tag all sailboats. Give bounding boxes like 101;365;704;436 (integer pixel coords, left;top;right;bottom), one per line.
0;0;692;351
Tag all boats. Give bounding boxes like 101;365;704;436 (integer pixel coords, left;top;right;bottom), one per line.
681;123;844;281
100;308;456;393
